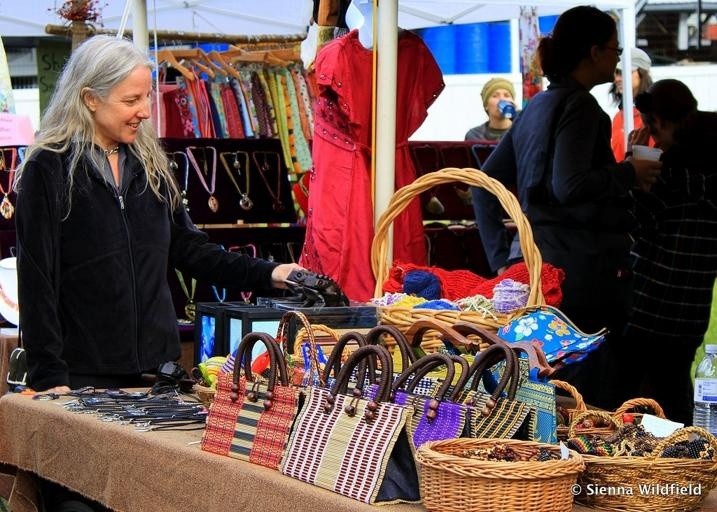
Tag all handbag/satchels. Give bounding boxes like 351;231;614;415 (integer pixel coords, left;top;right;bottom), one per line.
200;308;533;503
4;347;34;392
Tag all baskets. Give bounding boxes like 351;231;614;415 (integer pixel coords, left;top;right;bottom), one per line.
414;436;585;512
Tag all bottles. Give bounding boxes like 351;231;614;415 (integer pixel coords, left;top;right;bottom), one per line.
498;99;517;122
691;344;717;434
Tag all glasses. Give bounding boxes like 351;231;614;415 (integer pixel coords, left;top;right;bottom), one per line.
596;42;626;55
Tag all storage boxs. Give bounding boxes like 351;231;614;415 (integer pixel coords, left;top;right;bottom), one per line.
257;297;377;379
193;302;288;378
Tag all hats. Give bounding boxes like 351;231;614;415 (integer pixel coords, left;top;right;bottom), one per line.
498;308;603;370
615;46;652;74
480;77;515;109
637;79;693;109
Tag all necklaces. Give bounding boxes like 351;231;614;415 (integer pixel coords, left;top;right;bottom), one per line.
104;146;119;157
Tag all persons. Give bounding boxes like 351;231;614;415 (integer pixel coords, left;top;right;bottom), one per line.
11;33;312;512
460;4;717;430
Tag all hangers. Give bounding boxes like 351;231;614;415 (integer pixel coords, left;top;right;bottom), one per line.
153;40;302;80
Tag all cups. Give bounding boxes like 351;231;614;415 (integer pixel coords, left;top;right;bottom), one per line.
631;144;664;167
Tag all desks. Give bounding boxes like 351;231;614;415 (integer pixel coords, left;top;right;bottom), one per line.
0;386;717;512
